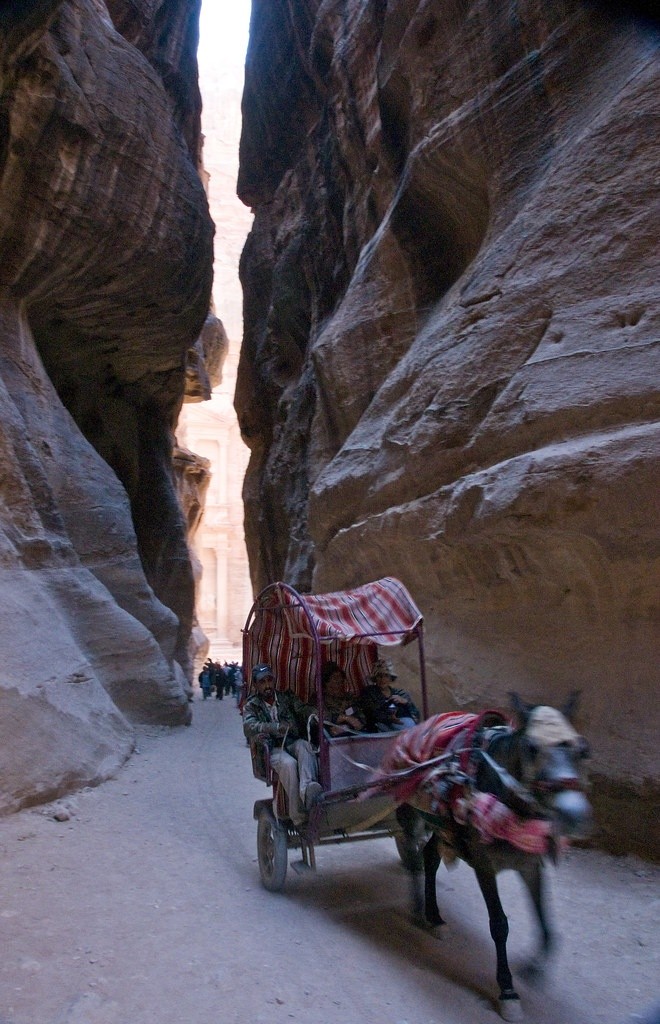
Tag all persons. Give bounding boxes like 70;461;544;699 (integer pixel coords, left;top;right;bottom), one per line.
307;660;369;737
357;659;421;734
198;657;243;700
242;664;323;827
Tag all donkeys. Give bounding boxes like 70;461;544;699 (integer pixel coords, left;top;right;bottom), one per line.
393;686;597;1023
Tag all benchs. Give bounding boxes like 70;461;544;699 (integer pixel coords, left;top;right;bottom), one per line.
250;734;279;786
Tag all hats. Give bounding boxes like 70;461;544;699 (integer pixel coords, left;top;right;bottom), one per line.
321;660;345;684
369;659;397;684
252;663;275;682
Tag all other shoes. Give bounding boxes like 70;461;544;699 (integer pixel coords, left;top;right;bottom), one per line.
306;781;322;814
297;823;309;838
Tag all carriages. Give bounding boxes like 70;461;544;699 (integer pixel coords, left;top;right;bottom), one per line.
240;581;594;1021
